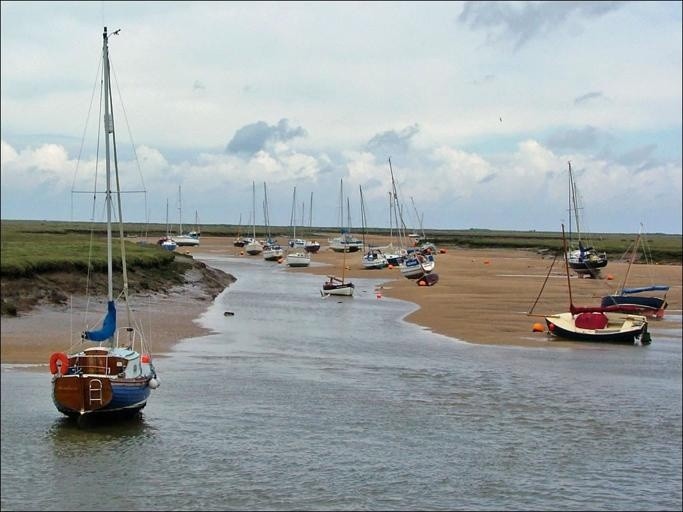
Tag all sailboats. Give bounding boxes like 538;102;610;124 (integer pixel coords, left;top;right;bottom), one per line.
49;24;158;418
233;156;435;297
156;185;200;252
544;160;668;347
